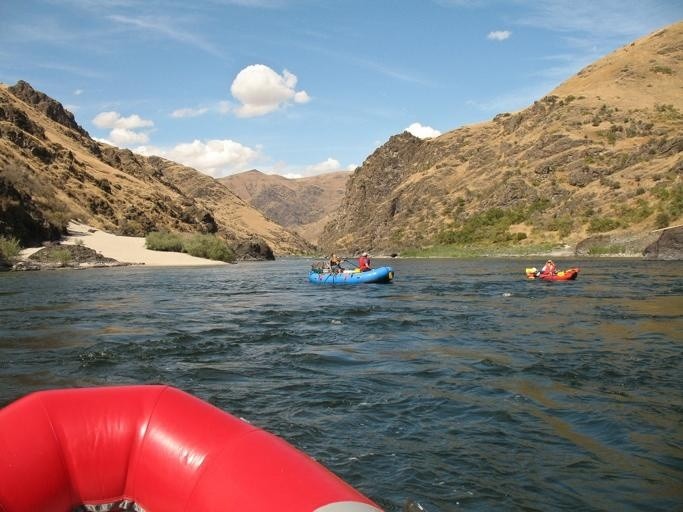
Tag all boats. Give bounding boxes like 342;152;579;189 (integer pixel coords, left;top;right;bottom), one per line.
307;266;394;286
1;386;383;512
524;266;579;280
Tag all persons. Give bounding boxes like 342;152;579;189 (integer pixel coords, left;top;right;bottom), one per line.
542;260;555;273
329;253;371;273
